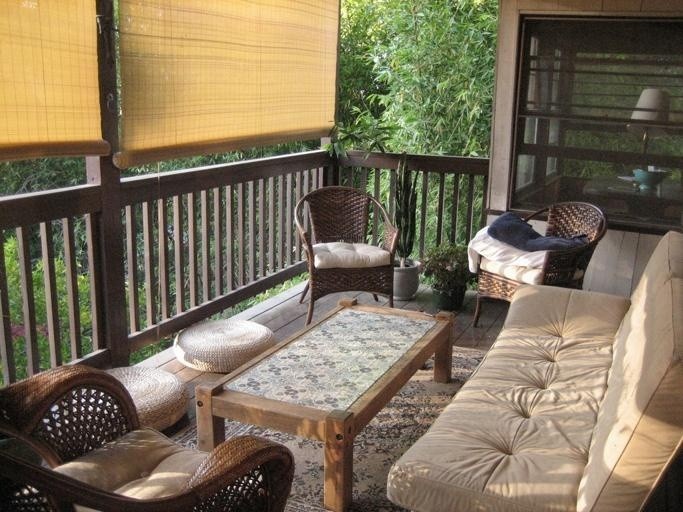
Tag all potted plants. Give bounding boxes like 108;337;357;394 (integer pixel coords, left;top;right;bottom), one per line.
387;144;421;300
419;244;469;310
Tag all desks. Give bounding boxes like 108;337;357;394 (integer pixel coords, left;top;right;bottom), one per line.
581;171;682;222
195;296;455;512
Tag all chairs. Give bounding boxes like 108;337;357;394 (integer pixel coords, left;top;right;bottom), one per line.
292;187;401;327
0;363;293;512
472;203;608;326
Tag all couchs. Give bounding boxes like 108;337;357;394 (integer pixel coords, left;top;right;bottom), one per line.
383;229;682;510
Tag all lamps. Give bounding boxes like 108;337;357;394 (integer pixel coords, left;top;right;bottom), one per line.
613;88;671;191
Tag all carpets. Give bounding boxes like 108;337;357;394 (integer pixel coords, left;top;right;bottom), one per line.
147;344;487;512
